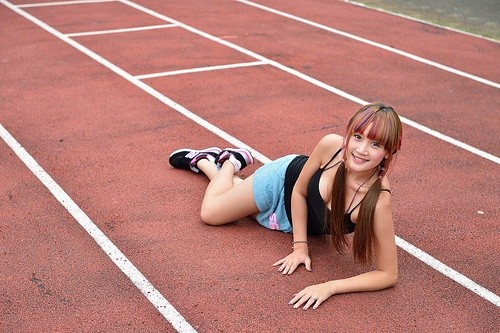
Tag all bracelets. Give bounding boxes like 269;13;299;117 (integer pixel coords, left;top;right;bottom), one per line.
292;241;309;245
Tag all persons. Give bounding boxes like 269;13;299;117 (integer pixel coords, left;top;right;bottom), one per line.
169;103;403;311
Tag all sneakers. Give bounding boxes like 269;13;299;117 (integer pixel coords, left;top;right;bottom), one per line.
214;147;255;173
168;146;223;174
